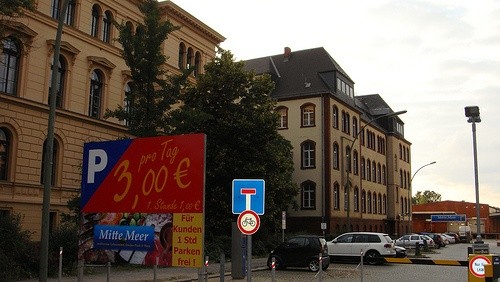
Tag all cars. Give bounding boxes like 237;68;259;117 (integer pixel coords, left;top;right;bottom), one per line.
266;234;330;272
391;231;461;259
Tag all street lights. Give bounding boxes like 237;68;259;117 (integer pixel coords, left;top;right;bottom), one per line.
465;106;486;243
345;109;407;233
407;161;436;234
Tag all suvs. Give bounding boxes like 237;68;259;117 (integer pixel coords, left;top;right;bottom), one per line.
325;231;397;266
